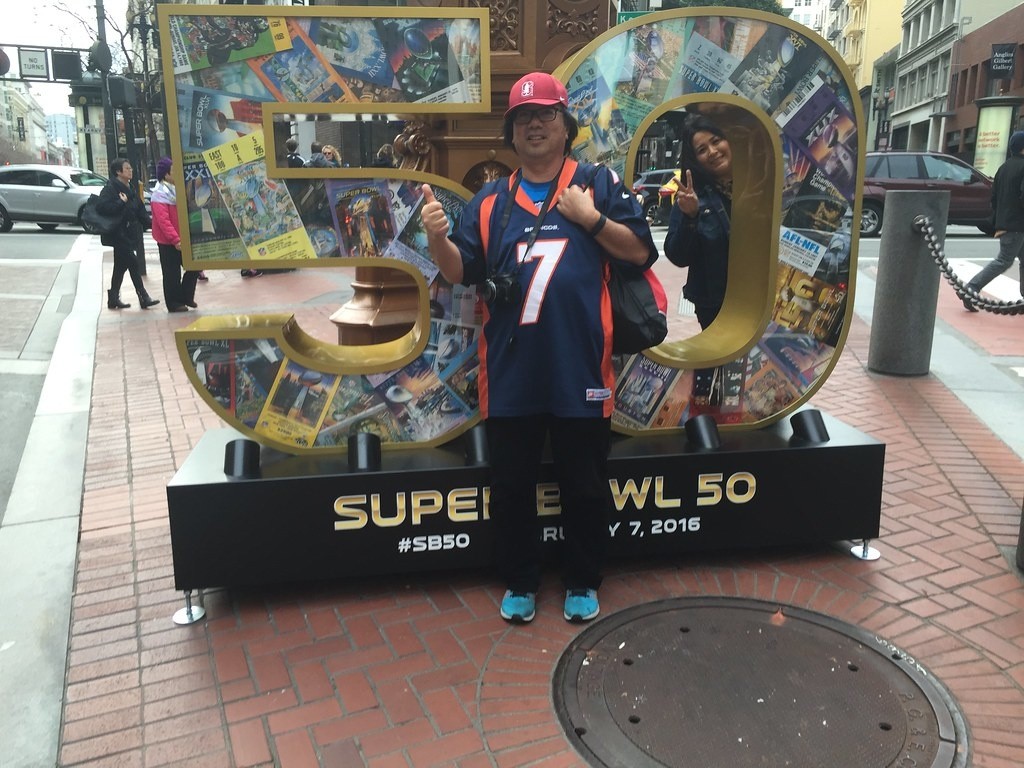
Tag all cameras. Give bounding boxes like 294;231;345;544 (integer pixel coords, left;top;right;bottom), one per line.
484;274;521;306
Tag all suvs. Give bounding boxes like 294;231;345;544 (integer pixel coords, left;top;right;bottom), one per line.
858;150;995;238
633;168;681;226
0;164;153;235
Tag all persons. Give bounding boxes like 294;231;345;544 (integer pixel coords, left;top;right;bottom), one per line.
198;268;263;281
420;74;659;621
287;139;306;168
152;158;200;312
302;142;335;168
97;159;159;309
322;145;341;167
955;132;1024;312
664;122;734;332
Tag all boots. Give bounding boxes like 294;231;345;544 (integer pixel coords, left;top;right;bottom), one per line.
107;289;130;308
137;289;159;309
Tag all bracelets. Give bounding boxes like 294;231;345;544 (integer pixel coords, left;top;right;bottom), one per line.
589;214;607;235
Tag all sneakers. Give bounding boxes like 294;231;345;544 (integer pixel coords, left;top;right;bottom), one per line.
500;590;539;622
564;588;600;623
197;272;208;282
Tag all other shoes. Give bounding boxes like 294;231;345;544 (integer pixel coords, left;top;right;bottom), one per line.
185;302;197;308
241;269;263;278
956;291;980;312
172;305;188;312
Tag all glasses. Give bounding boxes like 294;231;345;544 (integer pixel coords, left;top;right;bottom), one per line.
323;152;331;155
509;107;565;125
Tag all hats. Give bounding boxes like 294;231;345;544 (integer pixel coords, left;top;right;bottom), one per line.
504;72;568;117
1009;131;1024;154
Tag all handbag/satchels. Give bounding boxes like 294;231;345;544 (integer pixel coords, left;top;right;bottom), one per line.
583;161;668;356
80;181;126;236
